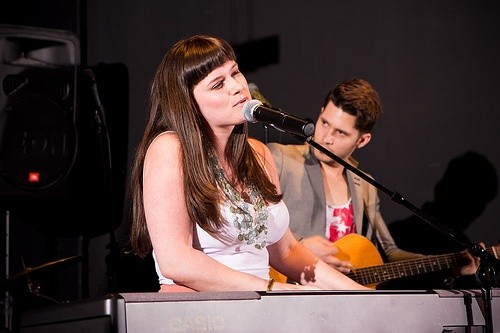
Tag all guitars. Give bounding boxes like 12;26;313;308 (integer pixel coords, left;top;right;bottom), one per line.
269;232;500;292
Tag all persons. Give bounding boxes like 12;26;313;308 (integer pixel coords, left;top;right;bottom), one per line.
267;80;486;276
130;35;372;292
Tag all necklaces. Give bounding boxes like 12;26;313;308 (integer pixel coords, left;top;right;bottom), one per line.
209;149;267;249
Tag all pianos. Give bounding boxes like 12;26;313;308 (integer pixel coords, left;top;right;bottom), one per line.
23;288;500;333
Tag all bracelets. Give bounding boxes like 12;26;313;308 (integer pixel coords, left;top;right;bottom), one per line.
267;279;275;291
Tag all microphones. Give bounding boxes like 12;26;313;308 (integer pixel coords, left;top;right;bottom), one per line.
83;68;105;121
242;99;314;136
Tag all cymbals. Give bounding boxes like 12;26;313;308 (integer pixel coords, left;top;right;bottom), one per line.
11;253;82;277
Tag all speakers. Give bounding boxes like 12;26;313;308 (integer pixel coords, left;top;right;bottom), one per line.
0;24;81;196
